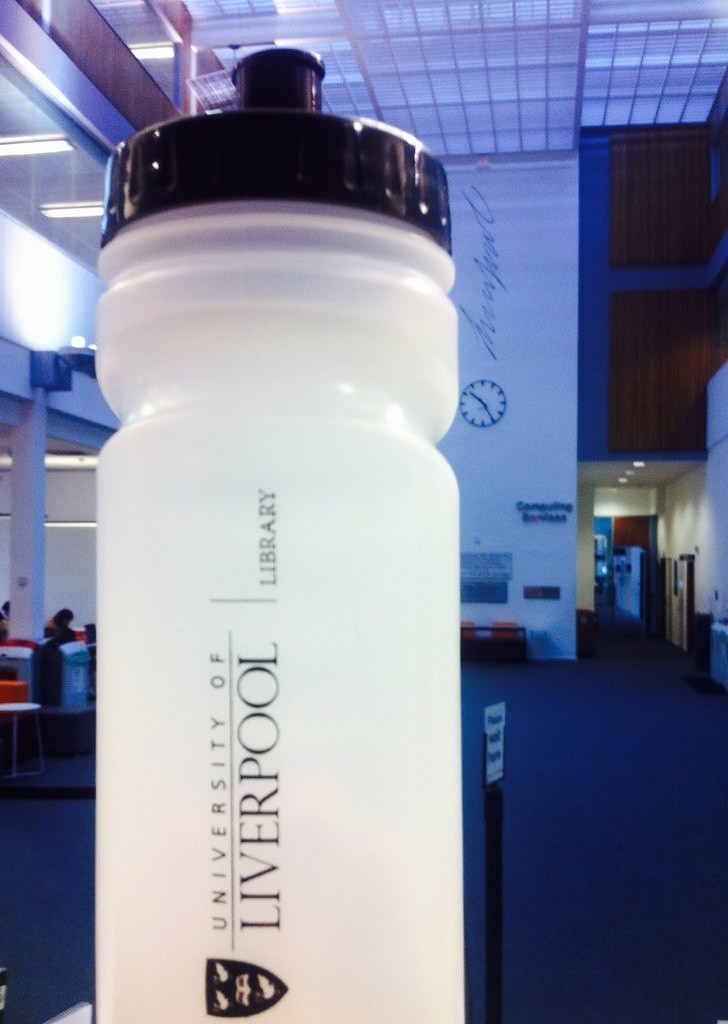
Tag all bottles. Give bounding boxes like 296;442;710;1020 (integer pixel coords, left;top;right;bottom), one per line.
93;48;465;1024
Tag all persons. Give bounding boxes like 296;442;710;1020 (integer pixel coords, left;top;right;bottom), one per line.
44;609;76;638
0;601;10;642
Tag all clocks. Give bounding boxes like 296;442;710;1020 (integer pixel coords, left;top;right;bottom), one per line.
459;379;506;428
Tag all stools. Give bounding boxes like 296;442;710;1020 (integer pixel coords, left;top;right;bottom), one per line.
0;703;45;780
